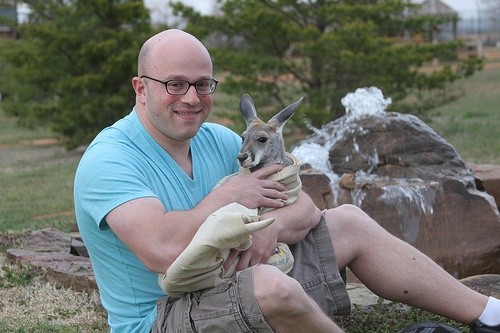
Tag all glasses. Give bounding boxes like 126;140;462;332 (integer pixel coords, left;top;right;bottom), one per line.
140;74;219;96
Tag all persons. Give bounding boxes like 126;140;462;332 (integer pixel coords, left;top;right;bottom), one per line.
73;29;500;332
